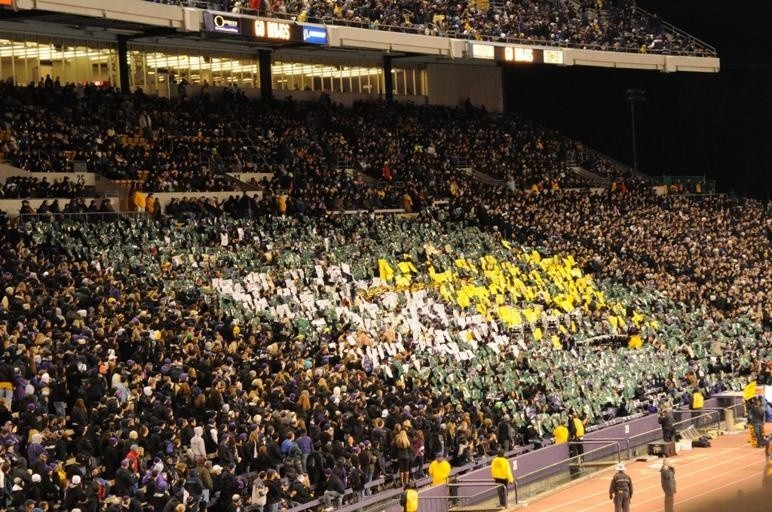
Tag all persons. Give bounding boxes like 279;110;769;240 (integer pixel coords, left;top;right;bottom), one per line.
2;72;772;511
148;1;721;57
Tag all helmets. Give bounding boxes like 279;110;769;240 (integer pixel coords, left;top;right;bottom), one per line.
616;463;625;471
